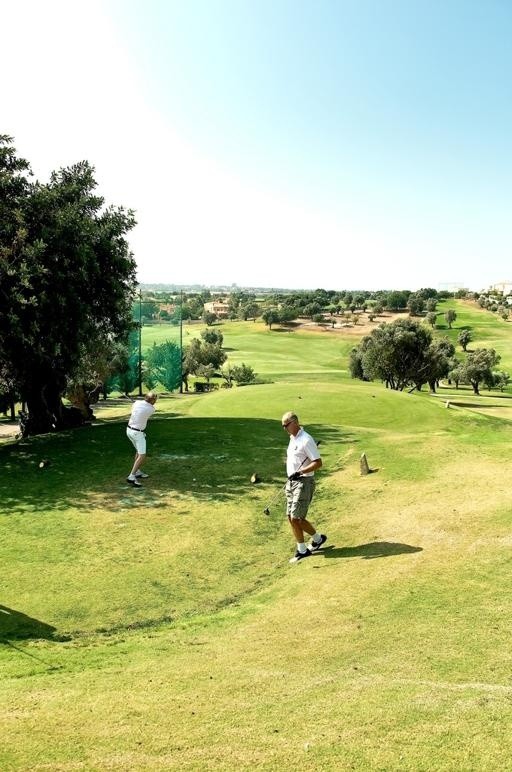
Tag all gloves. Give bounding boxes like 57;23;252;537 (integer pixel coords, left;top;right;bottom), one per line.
288;472;300;481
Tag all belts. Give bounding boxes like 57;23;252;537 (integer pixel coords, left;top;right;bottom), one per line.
127;425;144;432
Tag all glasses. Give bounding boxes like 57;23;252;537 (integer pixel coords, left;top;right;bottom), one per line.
283;419;296;427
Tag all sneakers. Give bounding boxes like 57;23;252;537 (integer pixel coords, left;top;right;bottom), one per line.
126;472;149;486
289;535;326;562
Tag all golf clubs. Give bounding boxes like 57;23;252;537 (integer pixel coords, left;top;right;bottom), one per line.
264;441;322;516
110;386;135;403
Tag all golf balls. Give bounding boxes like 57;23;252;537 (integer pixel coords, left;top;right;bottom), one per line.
39;463;44;467
193;478;196;481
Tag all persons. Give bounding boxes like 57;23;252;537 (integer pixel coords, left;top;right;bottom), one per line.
126;391;158;487
282;411;326;565
